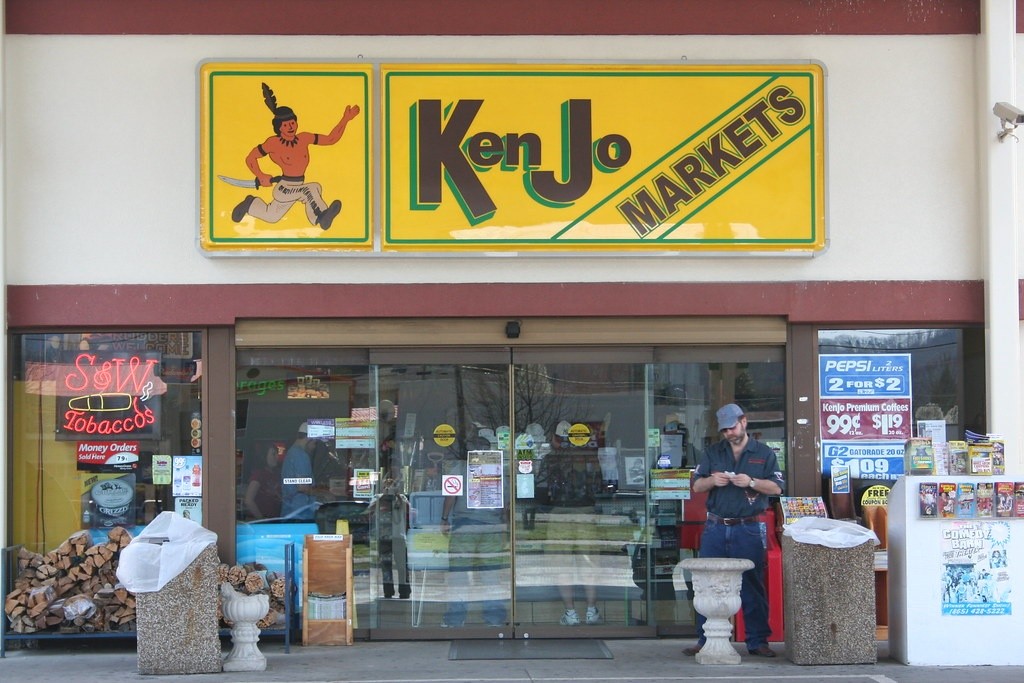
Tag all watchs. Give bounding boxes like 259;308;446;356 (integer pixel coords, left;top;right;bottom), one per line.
747;477;756;490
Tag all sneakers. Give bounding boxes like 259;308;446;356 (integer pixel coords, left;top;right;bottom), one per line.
560;612;582;626
586;609;603;625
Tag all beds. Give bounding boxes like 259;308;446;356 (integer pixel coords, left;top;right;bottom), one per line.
0;540;297;658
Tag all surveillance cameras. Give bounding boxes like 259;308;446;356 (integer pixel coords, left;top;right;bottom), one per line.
993;101;1024;125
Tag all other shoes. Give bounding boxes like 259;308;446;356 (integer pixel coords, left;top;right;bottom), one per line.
749;646;777;657
681;646;701;656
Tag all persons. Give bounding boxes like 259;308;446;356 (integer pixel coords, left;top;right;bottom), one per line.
360;479;413;599
633;420;704;597
684;403;783;660
243;416;604;624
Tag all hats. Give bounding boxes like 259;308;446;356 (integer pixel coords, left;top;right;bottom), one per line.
297;422;308;433
716;404;744;432
555;420;576;437
524;422;545;442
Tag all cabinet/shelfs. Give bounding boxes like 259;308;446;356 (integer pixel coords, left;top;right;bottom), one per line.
886;475;1024;668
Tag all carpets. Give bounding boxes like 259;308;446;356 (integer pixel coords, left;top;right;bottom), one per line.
448;638;615;659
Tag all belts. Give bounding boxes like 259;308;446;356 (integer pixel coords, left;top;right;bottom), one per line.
708;515;756;525
